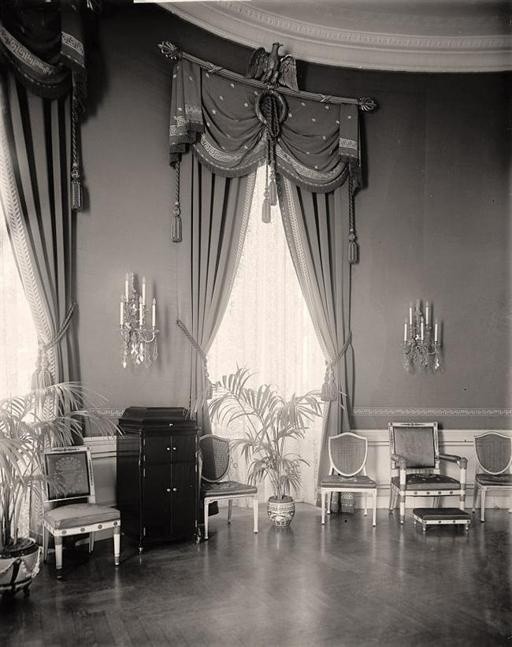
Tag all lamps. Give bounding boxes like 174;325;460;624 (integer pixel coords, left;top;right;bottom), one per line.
119;271;159;370
403;298;441;377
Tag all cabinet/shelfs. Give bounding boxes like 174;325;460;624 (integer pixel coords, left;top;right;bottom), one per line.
116;405;200;549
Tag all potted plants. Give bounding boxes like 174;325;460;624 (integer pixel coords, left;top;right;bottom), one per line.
0;383;123;601
192;364;324;530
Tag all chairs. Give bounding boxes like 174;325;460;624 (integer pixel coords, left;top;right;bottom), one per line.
199;434;260;540
320;432;379;529
387;422;468;525
42;445;121;572
471;432;512;523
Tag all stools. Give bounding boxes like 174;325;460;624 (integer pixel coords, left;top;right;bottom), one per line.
413;509;471;537
